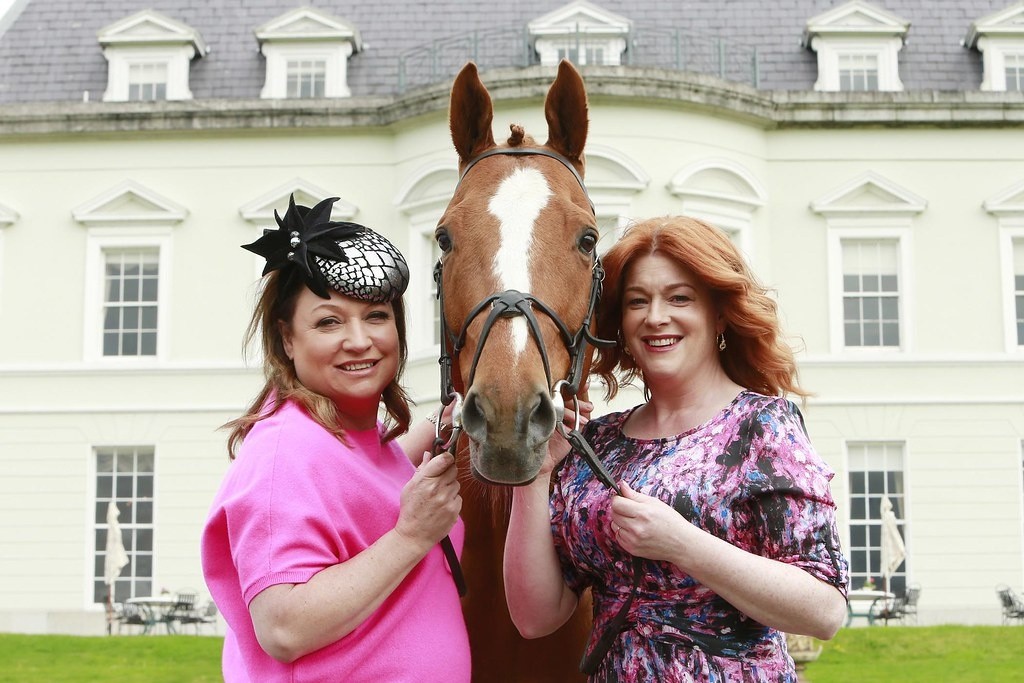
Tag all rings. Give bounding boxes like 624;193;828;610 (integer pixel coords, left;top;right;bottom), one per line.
615;525;621;540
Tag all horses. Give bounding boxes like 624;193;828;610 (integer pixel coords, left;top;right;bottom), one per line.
433;58;604;683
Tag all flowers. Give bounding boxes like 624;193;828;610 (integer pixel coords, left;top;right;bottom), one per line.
863;578;875;586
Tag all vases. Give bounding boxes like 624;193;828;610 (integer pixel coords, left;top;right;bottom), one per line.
862;585;876;590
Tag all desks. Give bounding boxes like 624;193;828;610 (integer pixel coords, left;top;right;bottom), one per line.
844;589;895;627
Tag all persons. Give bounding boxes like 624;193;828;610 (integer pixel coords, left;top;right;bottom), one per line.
504;213;854;683
201;199;474;683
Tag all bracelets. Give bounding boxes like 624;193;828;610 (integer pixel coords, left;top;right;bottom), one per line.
424;413;452;438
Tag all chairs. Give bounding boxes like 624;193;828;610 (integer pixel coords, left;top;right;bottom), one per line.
996;586;1024;626
99;590;218;636
875;597;907;626
896;588;921;627
